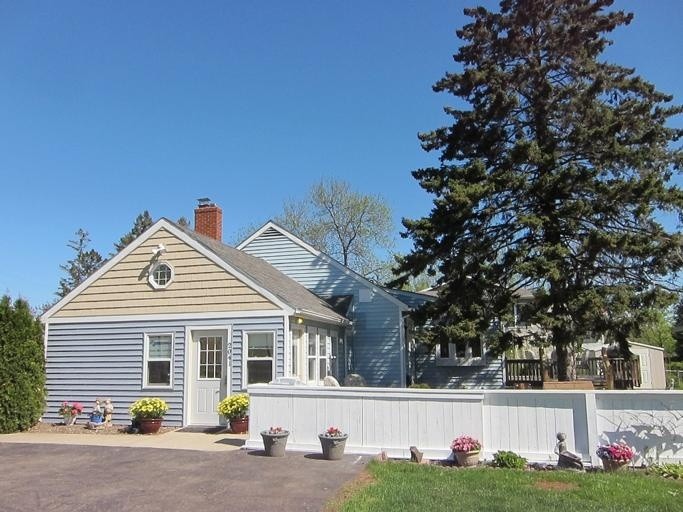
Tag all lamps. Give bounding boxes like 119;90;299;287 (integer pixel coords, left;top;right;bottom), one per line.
152;242;167;255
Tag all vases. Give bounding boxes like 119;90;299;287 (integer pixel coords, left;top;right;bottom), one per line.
63;414;78;426
316;434;348;461
229;414;248;434
259;428;291;458
602;459;631;472
135;417;163;434
453;449;480;469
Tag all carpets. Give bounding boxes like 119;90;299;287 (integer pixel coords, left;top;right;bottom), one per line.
174;424;227;436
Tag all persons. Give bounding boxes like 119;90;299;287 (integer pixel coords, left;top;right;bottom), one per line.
93;398;104;415
105;398;113;424
553;431;584;469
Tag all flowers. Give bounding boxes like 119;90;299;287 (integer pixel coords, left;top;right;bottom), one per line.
129;394;168;418
448;435;481;451
267;425;282;434
57;400;83;415
597;442;635;460
216;392;250;419
321;425;343;436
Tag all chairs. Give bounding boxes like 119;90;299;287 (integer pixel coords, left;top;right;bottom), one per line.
322;376;340;387
